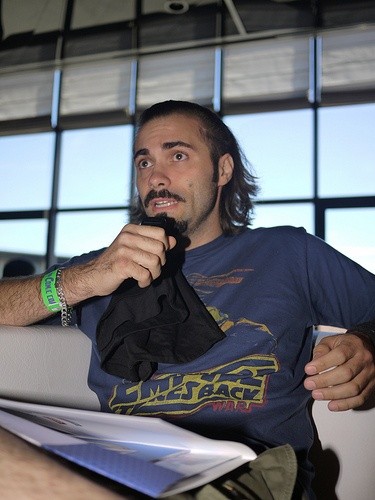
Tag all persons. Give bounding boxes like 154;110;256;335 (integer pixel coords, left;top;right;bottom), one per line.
0;99;374;500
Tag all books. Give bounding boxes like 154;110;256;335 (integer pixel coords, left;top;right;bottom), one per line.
0;396;257;499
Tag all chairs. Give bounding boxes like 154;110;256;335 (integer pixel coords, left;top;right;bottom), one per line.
0;318;375;500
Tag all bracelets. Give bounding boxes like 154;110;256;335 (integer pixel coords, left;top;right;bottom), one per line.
41;265;74;328
